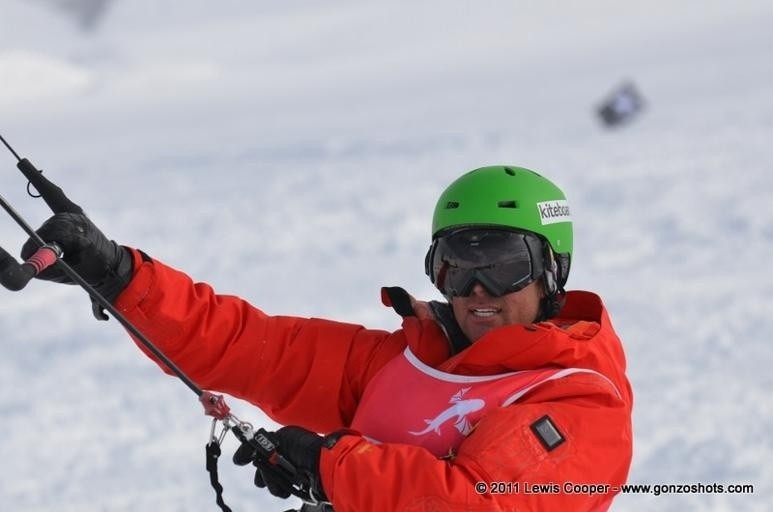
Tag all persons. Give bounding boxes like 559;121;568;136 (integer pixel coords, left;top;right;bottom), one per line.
19;165;636;512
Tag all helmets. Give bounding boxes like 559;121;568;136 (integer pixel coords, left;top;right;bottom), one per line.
425;166;574;297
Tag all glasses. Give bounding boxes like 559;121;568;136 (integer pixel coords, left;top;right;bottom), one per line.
429;228;545;296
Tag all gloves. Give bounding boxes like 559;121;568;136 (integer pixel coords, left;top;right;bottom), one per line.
21;211;135;322
232;425;325;505
